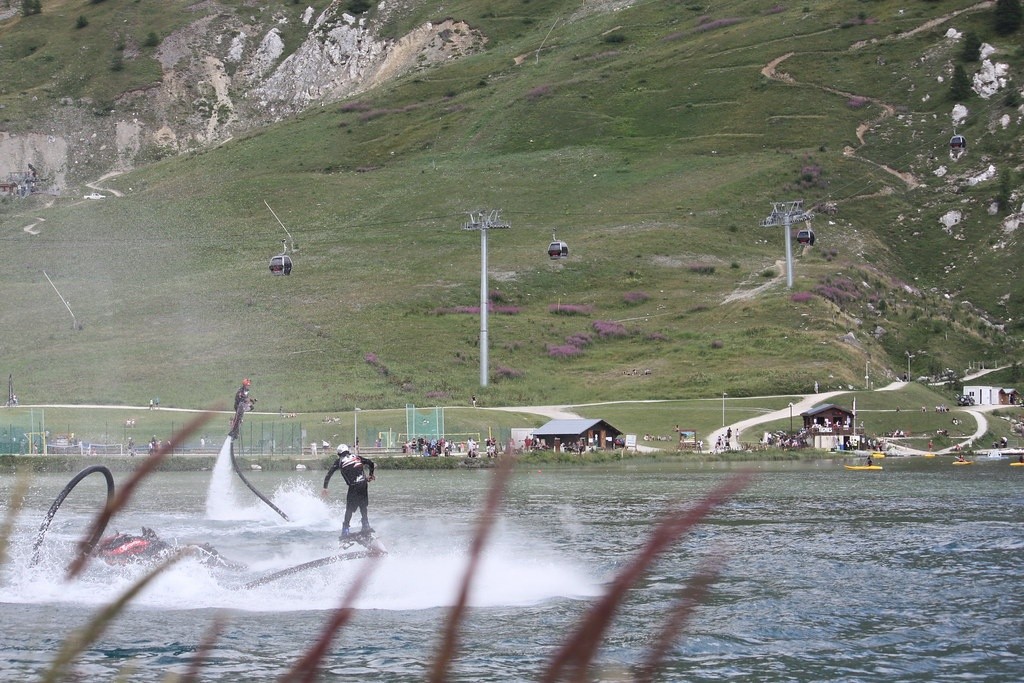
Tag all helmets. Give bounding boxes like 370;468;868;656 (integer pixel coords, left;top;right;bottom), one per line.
242;379;251;385
337;444;349;454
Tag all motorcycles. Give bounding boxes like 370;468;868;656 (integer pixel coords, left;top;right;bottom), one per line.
70;526;233;572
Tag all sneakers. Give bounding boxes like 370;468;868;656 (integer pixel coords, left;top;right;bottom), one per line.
342;525;350;535
361;522;370;531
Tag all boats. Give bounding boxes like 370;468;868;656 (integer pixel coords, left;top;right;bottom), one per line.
952;461;974;466
872;452;886;459
1009;462;1024;466
924;454;935;457
844;464;884;471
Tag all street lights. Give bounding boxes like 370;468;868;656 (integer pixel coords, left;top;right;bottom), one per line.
788;402;793;439
908;355;913;382
865;361;870;389
722;393;728;427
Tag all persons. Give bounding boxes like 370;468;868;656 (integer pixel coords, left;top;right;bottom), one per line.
11;393;18;404
353;436;360;452
146;395;161;412
45;429;50;443
310;440;319;460
19;439;26;455
69;431;99;457
372;372;705;475
124;432;179;461
230;377;256;436
198;433;215;448
705;354;1023;471
267;436;277;454
325;443;376;539
34;440;39;455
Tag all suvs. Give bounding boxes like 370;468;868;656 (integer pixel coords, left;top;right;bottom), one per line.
957;394;974;406
